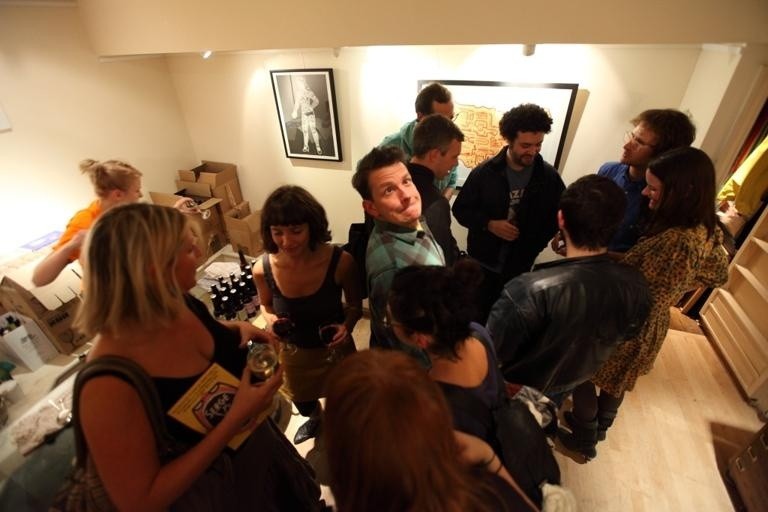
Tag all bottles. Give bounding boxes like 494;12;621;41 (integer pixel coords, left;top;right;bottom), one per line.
247;275;260;310
222;295;240;322
212;284;225;310
239;281;257;318
245;265;252;275
240;272;246;282
211;294;225;321
230;288;249;321
230;273;237;287
218;275;224;288
232;278;240;289
252;260;256;267
238;249;249;271
219;287;231;309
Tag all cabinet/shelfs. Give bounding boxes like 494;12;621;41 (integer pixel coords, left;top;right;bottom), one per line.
698;206;768;416
728;420;768;512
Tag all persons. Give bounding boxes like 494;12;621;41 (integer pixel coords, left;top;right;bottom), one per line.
481;175;649;439
32;157;141;289
451;104;566;284
380;81;462;205
405;115;466;268
248;184;364;446
350;147;446;351
385;260;559;512
70;202;333;511
292;77;323;154
551;109;697;258
319;350;540;512
557;147;729;463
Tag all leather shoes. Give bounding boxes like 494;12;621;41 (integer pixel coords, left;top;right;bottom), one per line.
293;419;318;444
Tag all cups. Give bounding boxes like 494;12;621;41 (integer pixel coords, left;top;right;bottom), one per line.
246;343;278;377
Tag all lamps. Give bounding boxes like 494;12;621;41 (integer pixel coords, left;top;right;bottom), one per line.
522;43;537;57
331;48;344;57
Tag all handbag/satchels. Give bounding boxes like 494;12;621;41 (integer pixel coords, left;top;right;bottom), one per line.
470;330;561;450
46;353;239;512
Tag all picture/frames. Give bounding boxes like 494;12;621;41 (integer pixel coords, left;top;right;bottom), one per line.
416;80;579;191
271;70;342;162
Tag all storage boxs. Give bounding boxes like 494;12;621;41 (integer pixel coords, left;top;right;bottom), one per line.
179;158;245;213
146;182;221;247
0;312;59;365
0;232;94;357
223;201;268;255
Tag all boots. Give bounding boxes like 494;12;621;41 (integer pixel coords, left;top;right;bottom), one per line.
554;414;599;465
564;406;618;443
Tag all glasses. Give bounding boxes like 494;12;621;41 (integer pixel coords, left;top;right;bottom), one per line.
623;130;657;153
381;317;406;332
